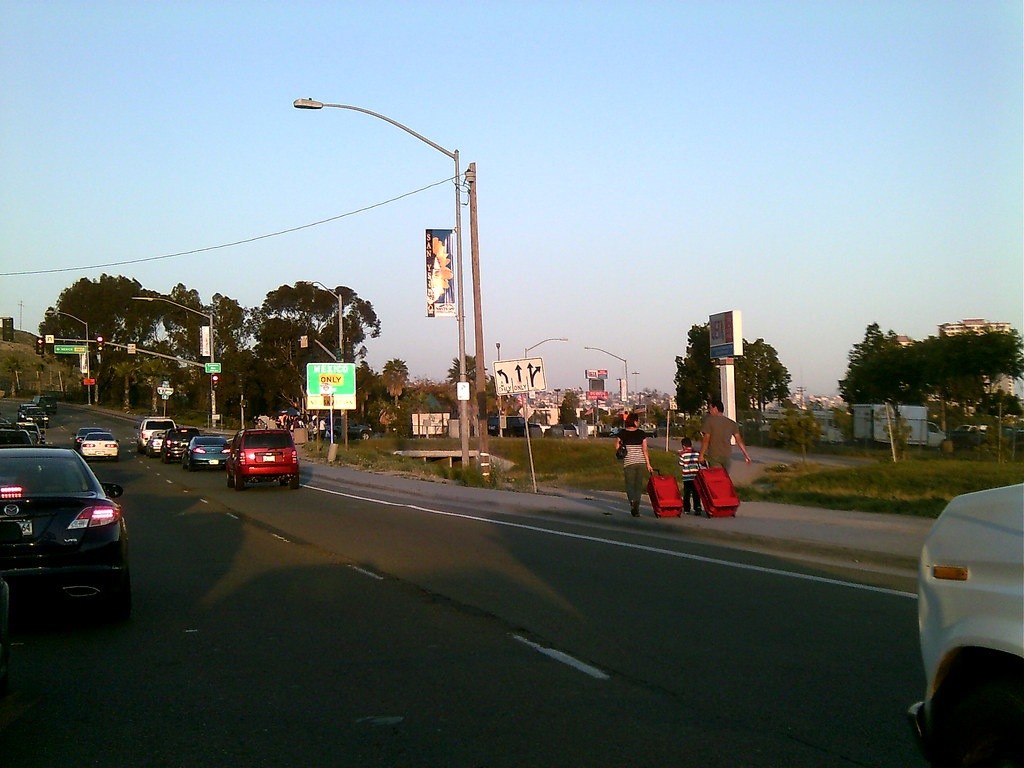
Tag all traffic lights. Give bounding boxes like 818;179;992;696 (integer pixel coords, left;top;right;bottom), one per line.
36;336;44;355
211;374;219;391
95;334;106;352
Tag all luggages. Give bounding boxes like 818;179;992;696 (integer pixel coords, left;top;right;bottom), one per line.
693;459;740;519
646;469;684;518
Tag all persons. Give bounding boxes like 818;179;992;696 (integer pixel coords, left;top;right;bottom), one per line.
678;437;703;516
698;400;751;473
252;410;328;441
616;413;655;517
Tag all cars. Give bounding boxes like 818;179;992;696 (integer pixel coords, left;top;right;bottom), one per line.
529;421;657;438
76;431;121;462
181;434;232;472
72;427;106;451
0;448;130;601
145;430;167;458
945;424;1024;453
0;403;50;449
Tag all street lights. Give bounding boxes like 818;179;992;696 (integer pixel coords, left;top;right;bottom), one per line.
132;296;216;430
293;96;470;473
554;389;561;407
45;310;91;406
632;372;639;403
524;338;570;359
585;347;629;415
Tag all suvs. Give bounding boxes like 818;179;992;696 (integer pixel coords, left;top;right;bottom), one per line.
31;395;58;415
319;416;374;441
157;428;202;464
133;416;181;455
220;427;300;490
487;416;526;438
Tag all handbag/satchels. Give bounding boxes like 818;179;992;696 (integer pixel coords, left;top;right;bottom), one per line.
615;443;628;460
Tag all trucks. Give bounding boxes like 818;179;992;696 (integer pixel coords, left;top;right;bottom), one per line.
852;403;948;450
